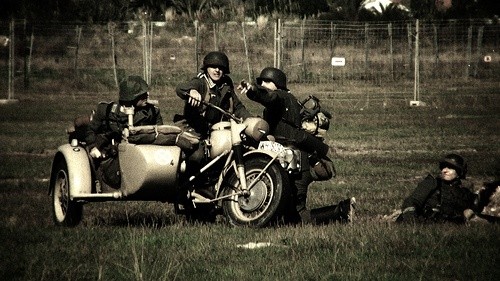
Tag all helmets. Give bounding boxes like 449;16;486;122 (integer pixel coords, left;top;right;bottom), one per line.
439;154;464;175
255;67;286;90
118;74;151;103
200;51;230;75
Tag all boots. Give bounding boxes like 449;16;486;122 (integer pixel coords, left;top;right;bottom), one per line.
310;197;356;225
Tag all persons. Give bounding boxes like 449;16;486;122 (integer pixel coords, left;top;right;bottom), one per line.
237;67;316;225
175;51;251;199
86;75;163;189
387;154;477;225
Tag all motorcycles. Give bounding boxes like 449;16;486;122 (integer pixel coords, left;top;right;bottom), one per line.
47;89;302;231
